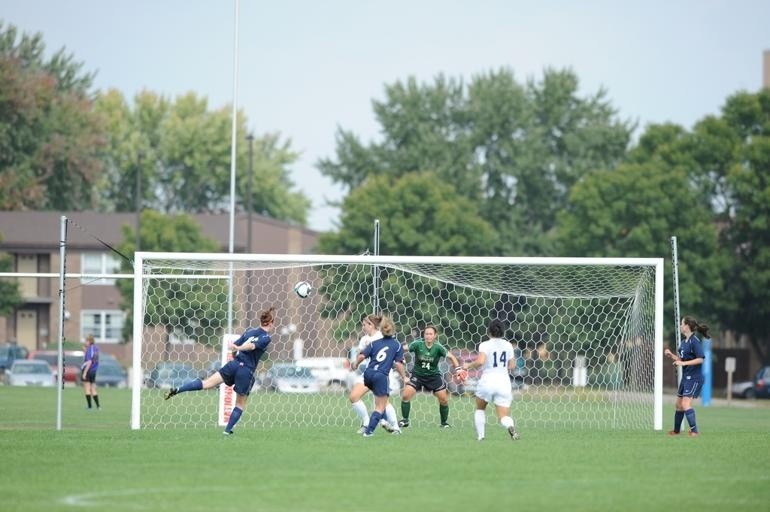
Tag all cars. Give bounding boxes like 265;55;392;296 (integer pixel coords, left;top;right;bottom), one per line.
732;381;753;400
297;357;403;395
95;357;128;390
5;361;56;390
0;342;29;368
30;350;85;383
151;363;201;393
445;350;484;396
271;362;319;393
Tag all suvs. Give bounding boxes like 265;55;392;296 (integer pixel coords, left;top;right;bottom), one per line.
753;365;770;399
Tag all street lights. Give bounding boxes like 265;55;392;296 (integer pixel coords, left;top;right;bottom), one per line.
245;125;257;338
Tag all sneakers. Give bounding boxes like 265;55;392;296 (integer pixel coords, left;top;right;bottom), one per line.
164;388;176;400
357;419;409;436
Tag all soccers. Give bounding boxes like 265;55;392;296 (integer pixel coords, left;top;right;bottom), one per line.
295;281;312;298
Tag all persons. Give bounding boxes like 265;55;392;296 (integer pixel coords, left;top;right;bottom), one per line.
344;313;521;441
163;309;277;435
664;314;709;435
80;332;101;412
